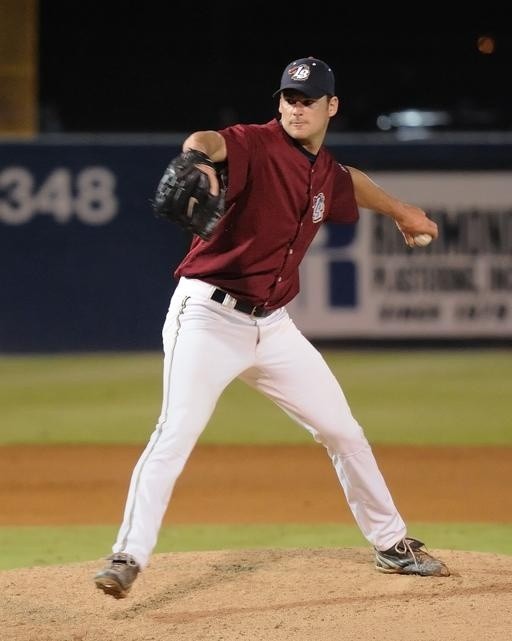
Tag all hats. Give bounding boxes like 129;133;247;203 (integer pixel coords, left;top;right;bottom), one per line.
271;56;335;99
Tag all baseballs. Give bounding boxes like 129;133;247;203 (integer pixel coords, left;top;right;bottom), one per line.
414;232;434;248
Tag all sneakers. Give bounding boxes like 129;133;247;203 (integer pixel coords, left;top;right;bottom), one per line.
374;537;450;577
93;552;141;599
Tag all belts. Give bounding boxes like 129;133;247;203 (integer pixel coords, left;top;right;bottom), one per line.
210;288;274;317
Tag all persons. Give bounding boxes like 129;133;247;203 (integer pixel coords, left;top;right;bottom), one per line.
95;56;452;601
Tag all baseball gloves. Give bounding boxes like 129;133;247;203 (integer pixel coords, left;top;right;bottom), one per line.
152;150;228;243
